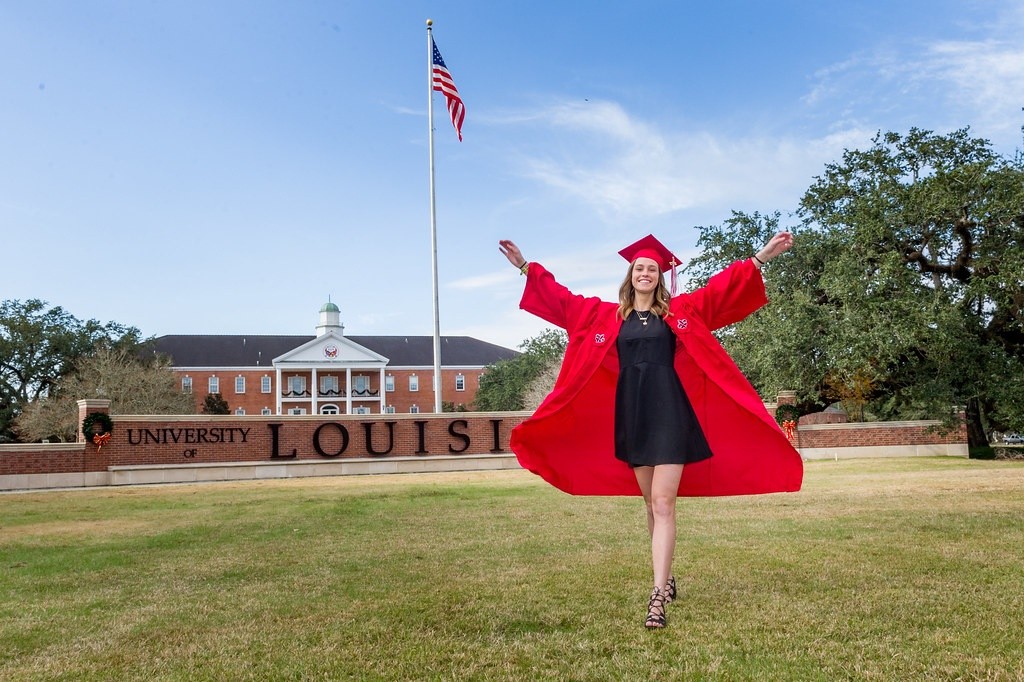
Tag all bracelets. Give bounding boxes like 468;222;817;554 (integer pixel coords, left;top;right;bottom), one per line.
520;263;529;275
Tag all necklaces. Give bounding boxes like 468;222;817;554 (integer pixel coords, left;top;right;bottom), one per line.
632;299;654;326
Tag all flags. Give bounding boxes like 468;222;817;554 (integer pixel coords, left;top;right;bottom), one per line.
432;32;466;143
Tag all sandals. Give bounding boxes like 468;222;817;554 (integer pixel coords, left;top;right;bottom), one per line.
660;575;676;602
644;587;666;629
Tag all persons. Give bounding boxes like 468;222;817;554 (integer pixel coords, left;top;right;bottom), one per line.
498;230;795;629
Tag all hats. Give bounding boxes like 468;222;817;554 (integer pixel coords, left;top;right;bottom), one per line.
617;235;682;297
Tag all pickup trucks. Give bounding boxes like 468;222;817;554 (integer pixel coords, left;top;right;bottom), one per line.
1002;434;1024;445
